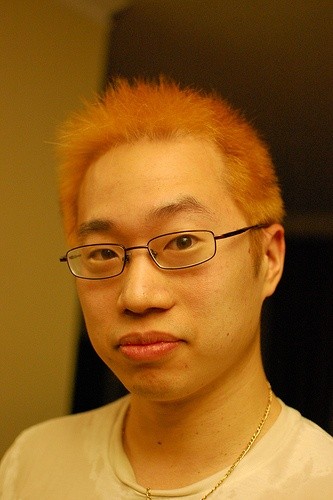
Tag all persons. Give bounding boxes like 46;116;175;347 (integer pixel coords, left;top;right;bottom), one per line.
0;78;332;500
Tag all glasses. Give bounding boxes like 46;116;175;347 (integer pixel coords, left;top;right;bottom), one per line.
59;223;269;280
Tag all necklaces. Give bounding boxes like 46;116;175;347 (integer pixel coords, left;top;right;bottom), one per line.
148;381;273;500
